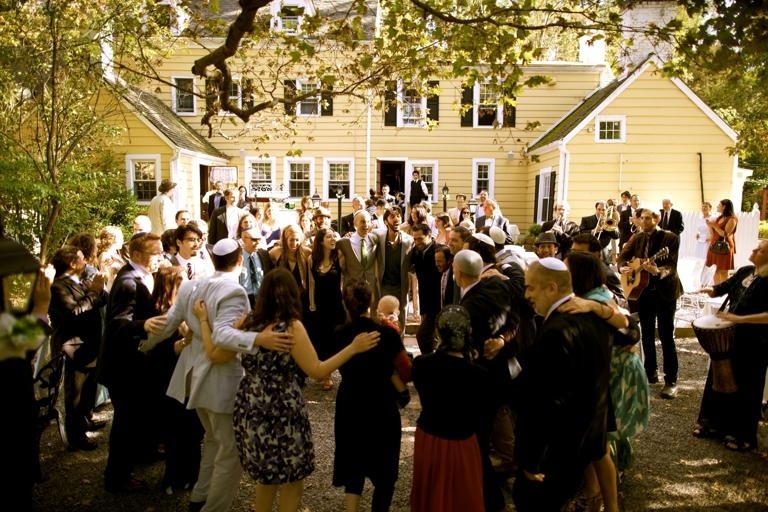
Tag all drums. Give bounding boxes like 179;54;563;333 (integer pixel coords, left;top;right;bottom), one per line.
692;314;734;391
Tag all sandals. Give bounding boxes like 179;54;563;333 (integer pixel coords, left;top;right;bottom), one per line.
723;436;758;453
322;376;334;390
691;426;725;440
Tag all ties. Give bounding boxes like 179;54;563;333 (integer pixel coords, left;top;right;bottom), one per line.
79;283;87;293
662;212;668;230
441;274;446;306
186;263;192;280
248;256;259;304
644;237;649;260
361;239;369;267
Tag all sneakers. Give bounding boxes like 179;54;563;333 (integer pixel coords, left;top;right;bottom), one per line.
105;478;148;497
89;419;107;431
660;383;679;398
70;437;98;452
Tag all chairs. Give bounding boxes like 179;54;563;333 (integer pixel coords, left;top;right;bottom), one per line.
33;352;66;480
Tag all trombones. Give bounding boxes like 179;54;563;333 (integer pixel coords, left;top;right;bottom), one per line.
592;198;621;241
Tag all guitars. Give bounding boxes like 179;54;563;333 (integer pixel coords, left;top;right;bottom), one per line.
621;246;670;301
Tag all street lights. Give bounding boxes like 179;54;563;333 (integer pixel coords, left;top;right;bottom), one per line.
312;189;321;209
442;182;449;212
334;186;345;234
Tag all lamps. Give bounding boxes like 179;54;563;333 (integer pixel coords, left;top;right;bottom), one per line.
239;147;245;157
507;150;514;161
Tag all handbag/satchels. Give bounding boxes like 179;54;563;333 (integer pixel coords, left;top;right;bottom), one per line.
709;230;732;256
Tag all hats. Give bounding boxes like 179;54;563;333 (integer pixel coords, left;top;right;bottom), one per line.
453;249;484;276
538;256;569;271
489;226;507;245
471;232;496;247
211;238;239;257
533;231;560;248
158;178;177;192
311;207;331;220
